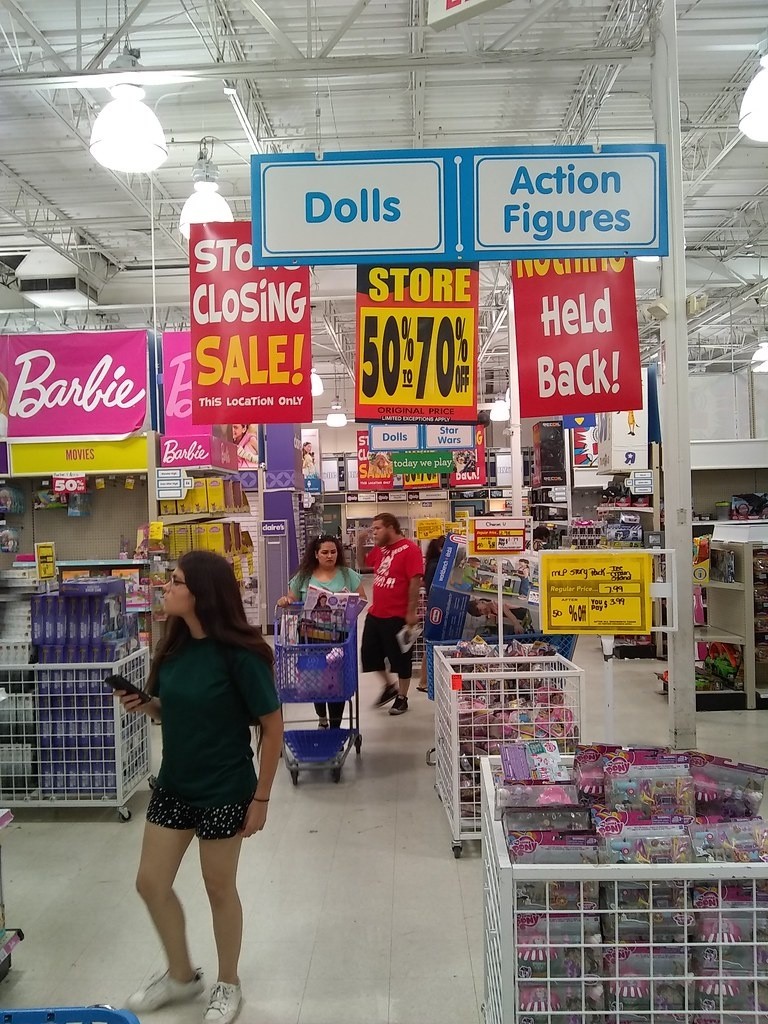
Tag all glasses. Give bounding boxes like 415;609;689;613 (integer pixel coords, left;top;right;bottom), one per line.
169;575;186;585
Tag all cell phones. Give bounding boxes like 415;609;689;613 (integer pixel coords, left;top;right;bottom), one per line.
105;675;151;707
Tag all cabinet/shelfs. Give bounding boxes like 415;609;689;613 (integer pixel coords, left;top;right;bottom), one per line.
0;473;149;650
305;488;534;571
145;432;250;657
530;441;756;711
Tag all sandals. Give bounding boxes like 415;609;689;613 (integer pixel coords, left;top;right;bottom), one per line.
417;684;428;692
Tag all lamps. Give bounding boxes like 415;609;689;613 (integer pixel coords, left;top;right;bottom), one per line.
646;295;669;319
87;0;170;174
309;366;322;395
489;355;511;421
177;137;234;243
752;308;767;371
325;361;348;428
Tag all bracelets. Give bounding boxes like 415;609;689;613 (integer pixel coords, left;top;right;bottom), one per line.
253;797;270;803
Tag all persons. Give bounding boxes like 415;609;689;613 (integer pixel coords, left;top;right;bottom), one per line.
533;525;553;550
466;596;532;634
232;424;259;469
416;536;445;694
114;552;284;1024
357;513;425;715
302;442;317;478
276;535;367;731
452;450;468;473
310;595;332;623
450;556;486;591
515;558;532;600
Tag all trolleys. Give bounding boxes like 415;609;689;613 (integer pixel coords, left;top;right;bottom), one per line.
422;635;580;766
272;601;362;786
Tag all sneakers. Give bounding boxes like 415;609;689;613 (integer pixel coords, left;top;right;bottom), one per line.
203;979;242;1024
388;694;408;714
375;683;399;708
126;967;204;1015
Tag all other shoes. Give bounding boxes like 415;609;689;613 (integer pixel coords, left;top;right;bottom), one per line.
318;723;329;730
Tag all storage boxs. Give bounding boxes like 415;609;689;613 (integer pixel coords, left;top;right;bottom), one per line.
158;473;250;596
532;419;567;485
28;565;147;791
